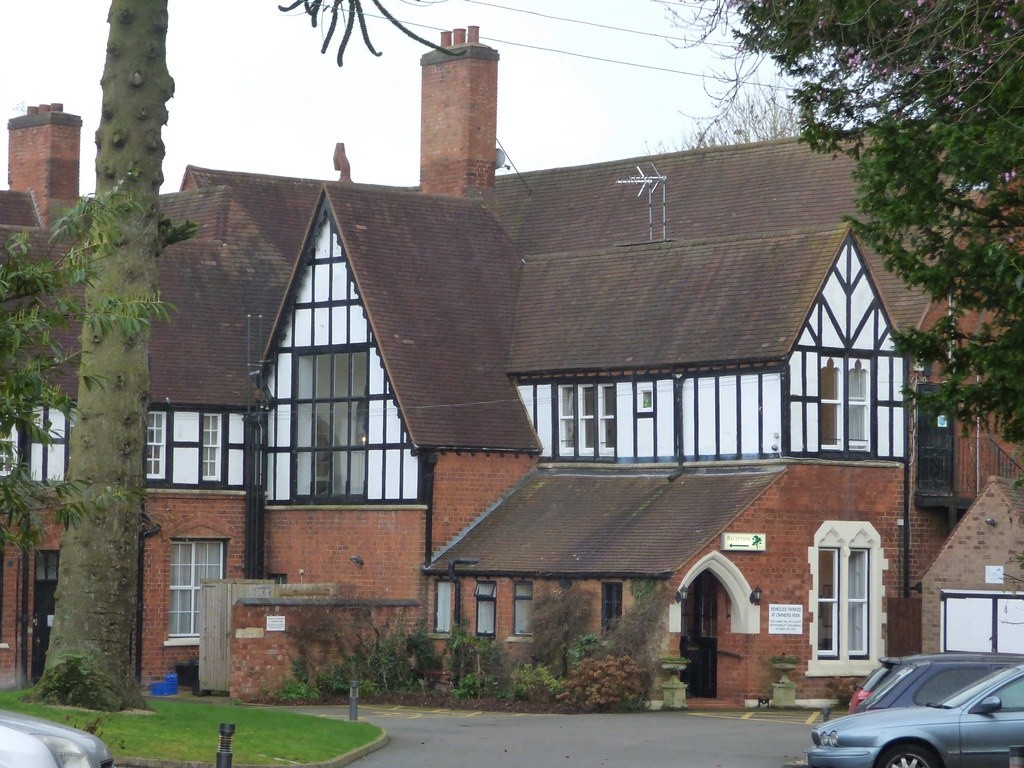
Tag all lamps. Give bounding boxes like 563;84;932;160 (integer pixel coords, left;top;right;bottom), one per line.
349;555;363;566
985;519;997;526
216;723;235;753
750;587;762;604
675;585;689;602
349;681;359;699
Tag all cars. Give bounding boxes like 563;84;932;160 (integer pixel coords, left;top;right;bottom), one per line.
848;654;1024;714
855;658;1019;712
1;709;116;768
805;661;1024;768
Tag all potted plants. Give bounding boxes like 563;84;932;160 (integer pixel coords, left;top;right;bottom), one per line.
660;656;691;710
772;653;799;708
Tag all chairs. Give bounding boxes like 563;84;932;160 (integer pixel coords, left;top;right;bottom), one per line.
148;673;178;695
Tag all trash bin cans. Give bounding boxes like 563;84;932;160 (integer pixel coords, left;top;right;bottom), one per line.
176;656;211;696
